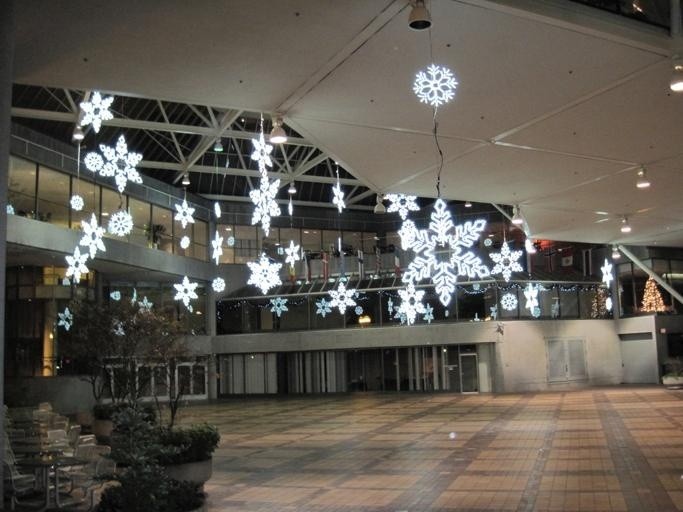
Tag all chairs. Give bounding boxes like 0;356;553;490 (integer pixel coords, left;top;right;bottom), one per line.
0;428;111;512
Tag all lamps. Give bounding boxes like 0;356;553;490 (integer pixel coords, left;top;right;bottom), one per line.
489;205;523;283
374;194;386;214
174;185;196;229
407;0;432;31
248;169;282;238
72;141;85;210
250;117;274;172
99;133;146;194
288;183;297;194
73;123;85;140
212;154;221;219
397;104;492;308
80;172;106;260
383;193;421;220
213;137;225;152
620;218;631;232
510;206;523;225
669;56;683;93
83;152;105;171
108;191;134;237
78;116;90;128
226;224;231;231
464;200;472;207
331;165;346;213
78;91;114;133
270;118;287;143
635;168;649;189
287;195;293;216
182;172;191;185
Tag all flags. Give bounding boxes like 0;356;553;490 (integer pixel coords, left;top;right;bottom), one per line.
559;247;575;273
526;241;537;274
542;244;557;273
286;248;401;283
581;249;593;276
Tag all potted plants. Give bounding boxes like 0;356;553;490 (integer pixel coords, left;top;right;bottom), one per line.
58;299;228;485
143;222;168;249
